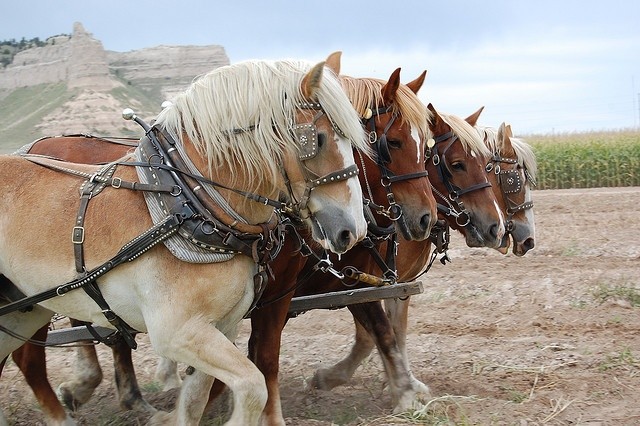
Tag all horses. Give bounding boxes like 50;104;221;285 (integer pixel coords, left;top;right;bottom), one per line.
109;100;506;417
0;50;367;426
152;118;540;407
10;66;439;426
